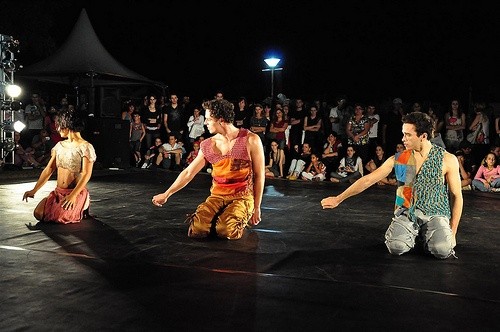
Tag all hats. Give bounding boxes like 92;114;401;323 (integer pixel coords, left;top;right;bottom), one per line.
393;98;402;104
193;141;200;144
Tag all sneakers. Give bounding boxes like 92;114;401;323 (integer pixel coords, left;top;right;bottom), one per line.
463;185;471;190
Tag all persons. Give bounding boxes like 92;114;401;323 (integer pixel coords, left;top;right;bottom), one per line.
151;99;265;240
320;111;463;259
1;91;499;195
23;106;97;223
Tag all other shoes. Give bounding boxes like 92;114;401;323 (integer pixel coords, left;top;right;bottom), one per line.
330;178;335;182
491;188;500;192
302;177;307;180
286;175;297;180
141;163;147;168
